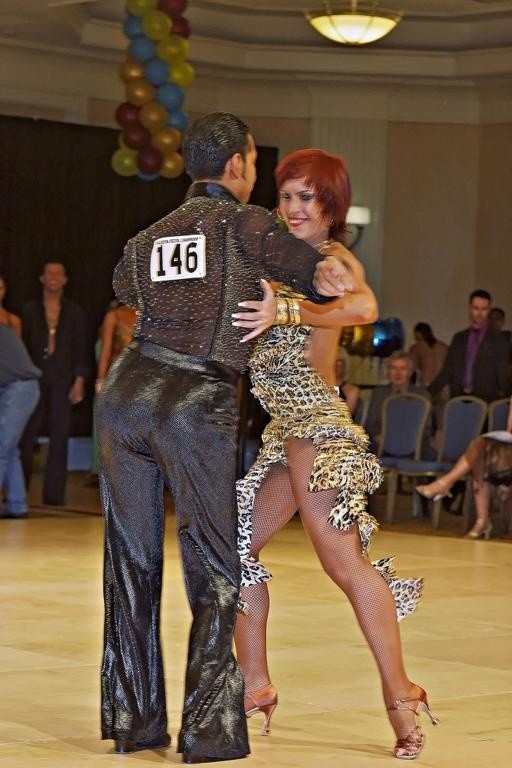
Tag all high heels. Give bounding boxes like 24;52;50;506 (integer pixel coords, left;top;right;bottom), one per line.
416;479;449;503
238;683;278;735
463;517;492;541
386;682;439;760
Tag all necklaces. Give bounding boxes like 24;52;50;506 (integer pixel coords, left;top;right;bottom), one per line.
48;327;56;336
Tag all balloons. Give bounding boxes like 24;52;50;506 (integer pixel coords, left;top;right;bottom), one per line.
112;1;193;181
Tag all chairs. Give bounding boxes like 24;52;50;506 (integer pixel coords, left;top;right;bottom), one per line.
364;392;432;520
394;395;489;531
353;397;369;425
488;398;511;435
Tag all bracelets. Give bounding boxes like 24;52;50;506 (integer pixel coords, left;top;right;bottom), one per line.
276;299;300;329
94;381;104;394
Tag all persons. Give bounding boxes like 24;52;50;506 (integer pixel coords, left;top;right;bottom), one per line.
1;325;42;518
333;359;358;421
92;302;124;486
230;151;439;759
2;260;90;510
429;290;509;513
92;114;355;762
0;279;22;337
490;308;512;399
362;351;433;517
417;397;512;541
409;321;451;434
94;303;138;401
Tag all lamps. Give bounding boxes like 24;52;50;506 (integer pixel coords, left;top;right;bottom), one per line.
304;2;404;47
344;206;371;253
